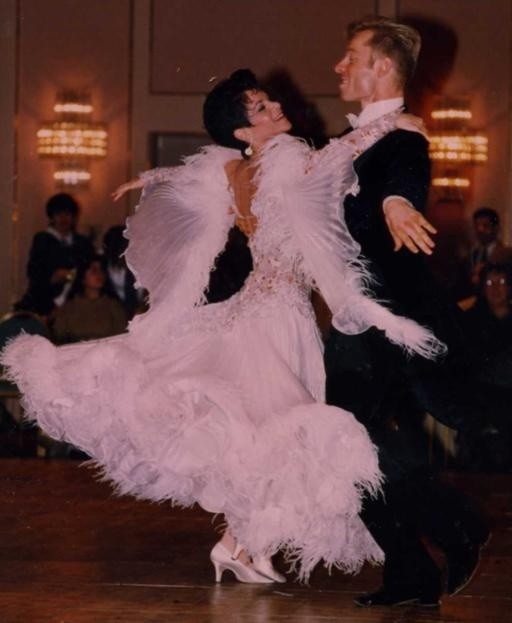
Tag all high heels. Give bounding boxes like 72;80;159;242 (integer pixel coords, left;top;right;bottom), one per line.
210;540;289;585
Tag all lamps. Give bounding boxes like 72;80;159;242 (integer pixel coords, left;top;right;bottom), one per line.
419;99;490;214
38;84;110;198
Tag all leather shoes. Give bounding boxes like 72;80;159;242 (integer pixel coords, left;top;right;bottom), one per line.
353;578;442;609
444;518;492;597
